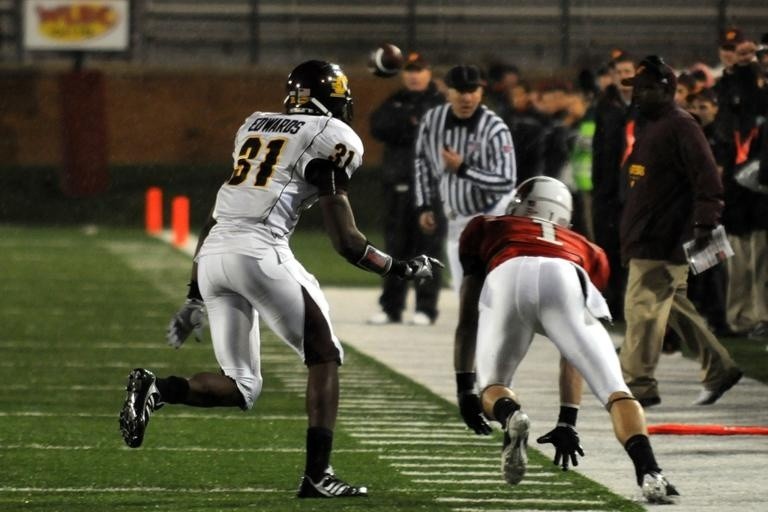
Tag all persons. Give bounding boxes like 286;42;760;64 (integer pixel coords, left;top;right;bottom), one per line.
619;61;743;407
369;53;517;332
118;60;448;500
451;172;682;505
484;27;767;341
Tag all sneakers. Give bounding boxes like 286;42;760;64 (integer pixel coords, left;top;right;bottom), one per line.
411;311;431;326
637;469;681;505
120;368;165;448
368;312;394;325
296;464;368;498
500;405;530;487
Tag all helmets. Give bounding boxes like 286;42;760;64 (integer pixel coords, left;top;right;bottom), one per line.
504;175;576;229
281;59;354;126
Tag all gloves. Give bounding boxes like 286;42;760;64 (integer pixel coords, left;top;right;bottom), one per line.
458;391;493;435
406;254;445;289
164;297;205;351
536;423;585;471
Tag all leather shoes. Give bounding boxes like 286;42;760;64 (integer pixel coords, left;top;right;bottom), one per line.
638;396;661;407
691;367;745;405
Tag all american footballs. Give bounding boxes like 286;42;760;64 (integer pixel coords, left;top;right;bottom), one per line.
367;45;402;81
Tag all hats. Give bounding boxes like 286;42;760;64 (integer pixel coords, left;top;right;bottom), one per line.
443;65;486;92
398;51;429;72
720;29;744;50
686;87;718;104
621;63;677;94
